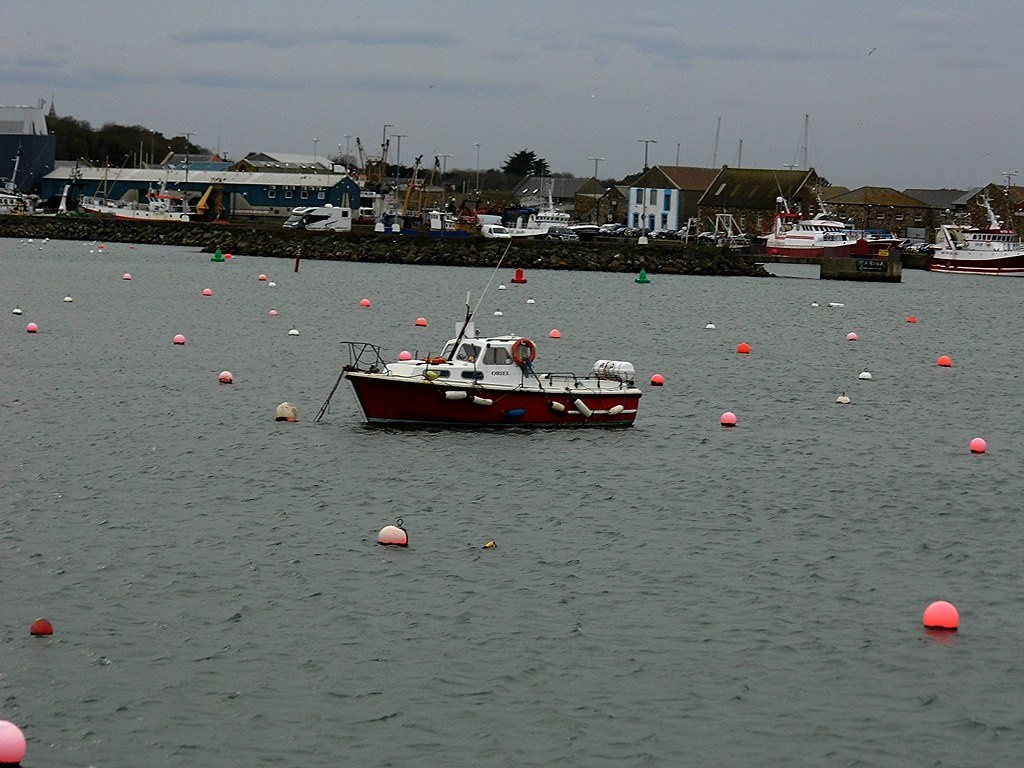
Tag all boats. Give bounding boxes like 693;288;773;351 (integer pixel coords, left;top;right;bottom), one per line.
369;128;502;234
761;192;933;280
0;156;34;215
504;178;570;239
339;241;642;428
79;141;223;222
929;170;1024;277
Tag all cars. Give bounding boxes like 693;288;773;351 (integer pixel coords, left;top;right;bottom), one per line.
596;222;753;247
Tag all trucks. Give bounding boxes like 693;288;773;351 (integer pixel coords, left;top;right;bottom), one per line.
283;204;351;237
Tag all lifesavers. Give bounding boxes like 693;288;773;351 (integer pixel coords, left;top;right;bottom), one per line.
512;338;536;365
422;356;446;364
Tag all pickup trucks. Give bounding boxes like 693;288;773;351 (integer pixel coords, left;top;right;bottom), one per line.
481;225;512;238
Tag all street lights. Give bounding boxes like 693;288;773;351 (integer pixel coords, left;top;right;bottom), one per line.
474;141;481;194
588;156;605;203
344;134;354;206
